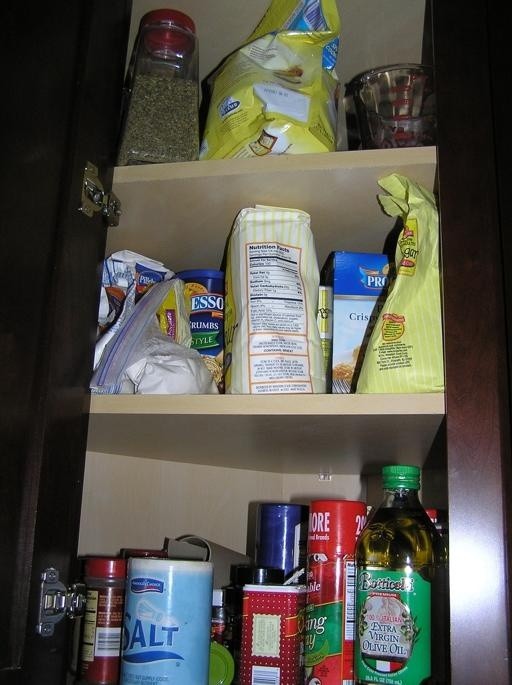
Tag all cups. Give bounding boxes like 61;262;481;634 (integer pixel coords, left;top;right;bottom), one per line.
349;64;436;148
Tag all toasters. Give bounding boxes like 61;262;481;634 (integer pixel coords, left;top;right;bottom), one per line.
117;7;200;166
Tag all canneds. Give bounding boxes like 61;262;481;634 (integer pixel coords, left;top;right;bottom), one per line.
163;270;226;395
119;555;214;685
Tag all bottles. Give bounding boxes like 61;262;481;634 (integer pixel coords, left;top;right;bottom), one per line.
212;564;283;641
354;466;444;684
76;556;127;683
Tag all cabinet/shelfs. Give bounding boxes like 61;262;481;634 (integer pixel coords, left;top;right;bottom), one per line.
72;142;446;681
